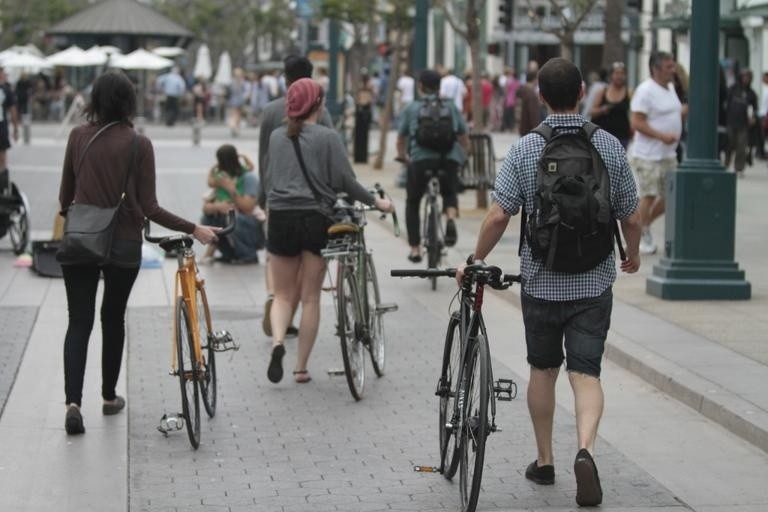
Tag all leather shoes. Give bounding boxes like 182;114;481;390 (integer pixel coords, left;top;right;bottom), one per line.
215;255;258;265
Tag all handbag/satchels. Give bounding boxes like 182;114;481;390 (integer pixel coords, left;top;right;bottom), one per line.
54;201;120;266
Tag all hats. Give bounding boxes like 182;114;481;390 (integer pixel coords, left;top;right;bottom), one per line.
286;77;324;118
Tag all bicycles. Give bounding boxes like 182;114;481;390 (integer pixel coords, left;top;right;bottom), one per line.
394;157;446;289
142;210;240;448
387;263;525;512
320;181;403;400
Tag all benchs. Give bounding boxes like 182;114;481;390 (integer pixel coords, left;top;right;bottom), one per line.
397;132;508;192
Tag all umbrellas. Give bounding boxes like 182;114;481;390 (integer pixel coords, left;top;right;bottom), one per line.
215;49;233;117
0;42;187;84
192;43;215;93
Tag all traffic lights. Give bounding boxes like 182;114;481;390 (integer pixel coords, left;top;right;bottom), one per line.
498;1;513;33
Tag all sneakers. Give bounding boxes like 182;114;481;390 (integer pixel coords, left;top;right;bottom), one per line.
65;406;86;434
574;449;602;506
525;459;555;484
196;256;215;267
407;252;421;262
444;219;457;246
102;396;125;415
638;227;658;255
262;295;298;338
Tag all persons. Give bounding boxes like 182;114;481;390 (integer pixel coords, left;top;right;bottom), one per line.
626;49;683;257
252;55;338;337
53;67;223;436
232;64;520;155
191;143;255;264
260;77;398;386
668;61;767;179
452;54;645;509
1;54;229;181
516;61;635;153
388;69;473;264
198;173;266;265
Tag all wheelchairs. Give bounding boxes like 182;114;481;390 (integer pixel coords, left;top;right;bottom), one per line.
0;167;31;255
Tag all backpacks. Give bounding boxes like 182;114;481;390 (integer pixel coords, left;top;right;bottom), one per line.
408;97;457;157
517;125;627;274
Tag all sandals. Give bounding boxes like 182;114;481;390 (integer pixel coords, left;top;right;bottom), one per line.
267;341;286;383
292;370;312;383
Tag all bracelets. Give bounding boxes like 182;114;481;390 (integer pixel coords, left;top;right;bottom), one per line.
231;189;239;198
466;254;475;266
